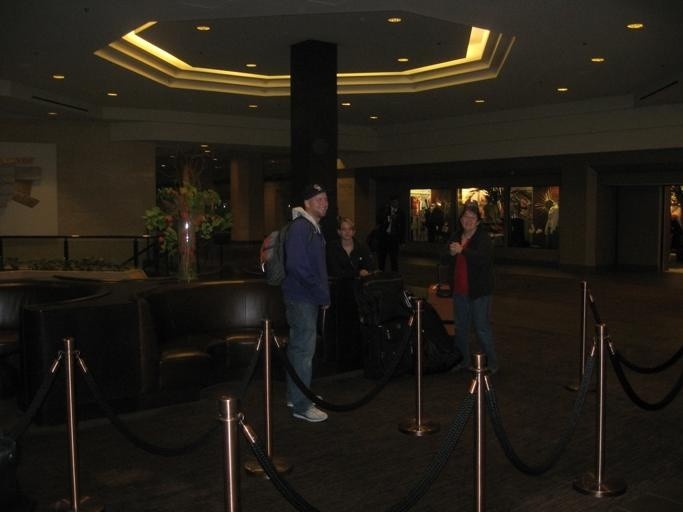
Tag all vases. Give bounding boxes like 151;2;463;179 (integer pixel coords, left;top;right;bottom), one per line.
177;220;198;281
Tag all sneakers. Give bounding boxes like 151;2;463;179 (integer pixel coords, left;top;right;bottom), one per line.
284;394;328;423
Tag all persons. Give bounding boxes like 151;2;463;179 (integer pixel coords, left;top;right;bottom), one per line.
326;215;369;372
440;204;498;378
269;181;333;424
374;193;408;273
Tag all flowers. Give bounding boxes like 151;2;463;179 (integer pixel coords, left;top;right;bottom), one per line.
141;184;235;277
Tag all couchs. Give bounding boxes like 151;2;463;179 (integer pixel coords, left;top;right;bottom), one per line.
3;271;342;426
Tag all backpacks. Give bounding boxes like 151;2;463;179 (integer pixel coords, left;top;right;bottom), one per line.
257;215;315;286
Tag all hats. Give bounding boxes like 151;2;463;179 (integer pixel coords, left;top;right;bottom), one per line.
298;180;326;201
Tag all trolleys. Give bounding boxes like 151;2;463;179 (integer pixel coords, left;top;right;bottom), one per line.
351;269;464;377
